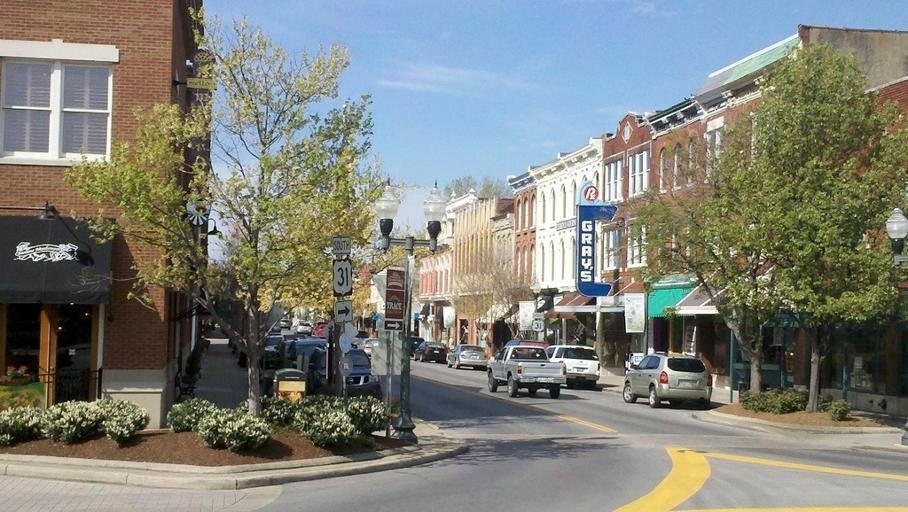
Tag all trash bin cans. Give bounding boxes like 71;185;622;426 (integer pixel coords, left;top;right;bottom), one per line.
273;368;308;401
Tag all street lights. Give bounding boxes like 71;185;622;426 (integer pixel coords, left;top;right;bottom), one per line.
375;177;444;445
885;207;908;266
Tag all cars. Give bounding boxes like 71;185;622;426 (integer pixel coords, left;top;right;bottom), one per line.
622;353;712;408
408;335;601;397
263;318;377;403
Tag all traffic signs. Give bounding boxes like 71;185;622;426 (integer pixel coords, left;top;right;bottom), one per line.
532;320;544;331
332;258;353;297
